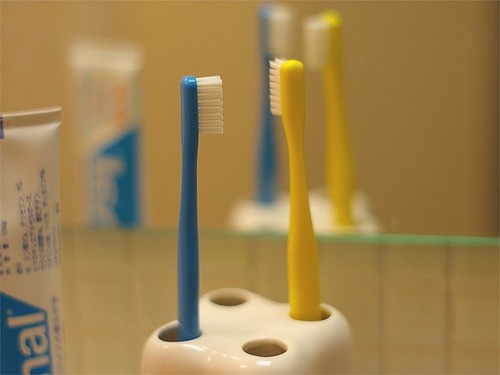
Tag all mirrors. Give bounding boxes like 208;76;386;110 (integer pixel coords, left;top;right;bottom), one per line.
1;1;500;246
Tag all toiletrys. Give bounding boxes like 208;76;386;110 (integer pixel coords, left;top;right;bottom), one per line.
1;105;67;374
64;42;144;233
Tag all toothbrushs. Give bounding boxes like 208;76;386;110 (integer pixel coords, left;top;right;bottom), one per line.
256;6;294;207
302;9;357;231
267;57;324;322
176;75;225;342
